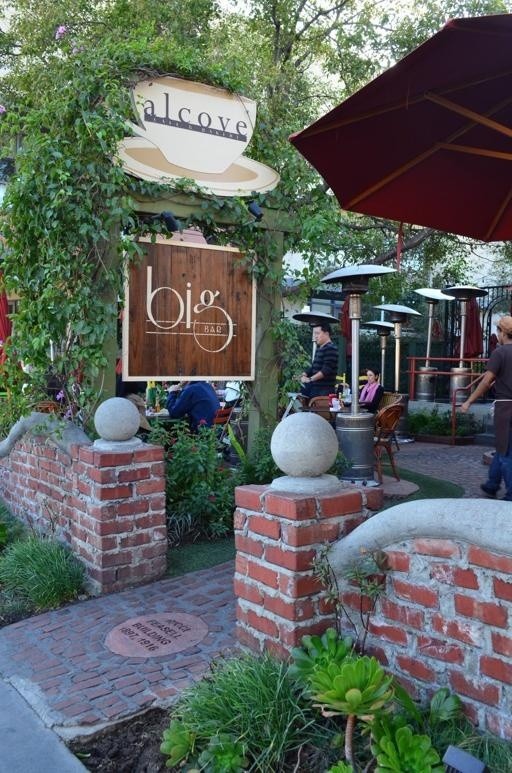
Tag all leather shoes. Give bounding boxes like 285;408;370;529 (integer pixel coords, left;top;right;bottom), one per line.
480;483;496;496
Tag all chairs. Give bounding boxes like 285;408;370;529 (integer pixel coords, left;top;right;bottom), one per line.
372;402;401;482
378;393;403;450
309;395;337;427
212;403;236;452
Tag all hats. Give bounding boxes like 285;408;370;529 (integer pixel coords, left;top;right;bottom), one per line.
497;315;512;335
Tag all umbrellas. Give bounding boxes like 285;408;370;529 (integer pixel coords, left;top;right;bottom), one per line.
288;12;511;242
453;297;483;397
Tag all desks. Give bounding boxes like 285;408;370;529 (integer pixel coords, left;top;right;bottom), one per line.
146;408;192;434
298;405;369;429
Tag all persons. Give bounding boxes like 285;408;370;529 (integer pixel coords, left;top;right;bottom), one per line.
460;315;512;500
167;381;220;427
299;322;338;396
116;373;168;399
225;381;242;407
358;366;385;413
